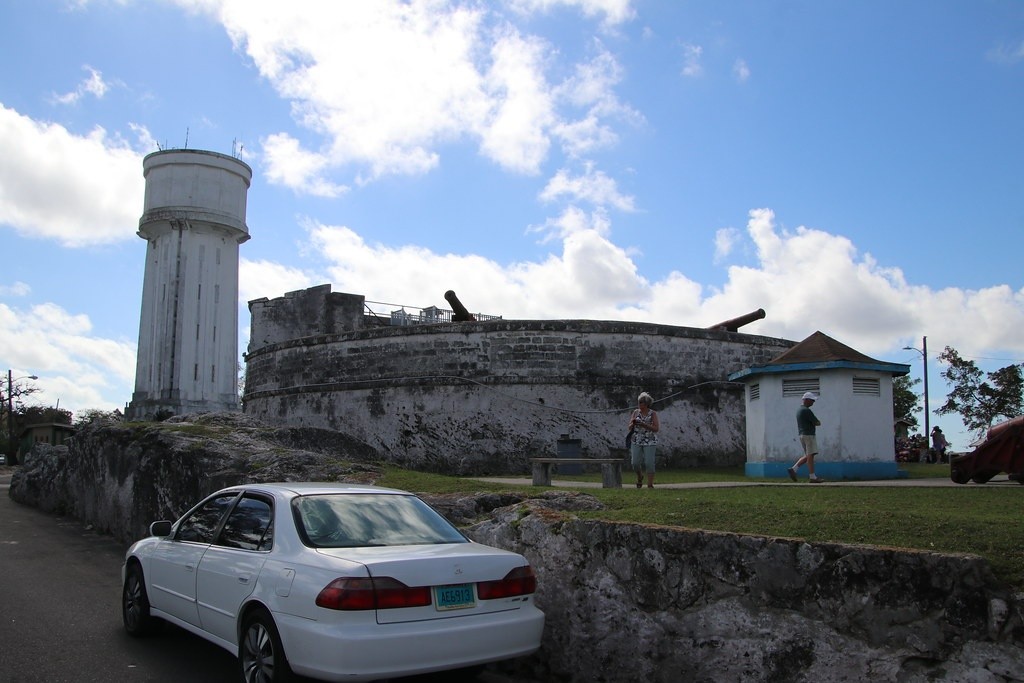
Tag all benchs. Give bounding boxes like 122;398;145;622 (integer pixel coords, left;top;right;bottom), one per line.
530;458;625;488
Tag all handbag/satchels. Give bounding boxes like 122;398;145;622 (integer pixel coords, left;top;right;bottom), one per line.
625;430;634;448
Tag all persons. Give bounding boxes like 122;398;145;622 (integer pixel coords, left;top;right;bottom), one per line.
787;392;825;483
629;392;659;490
930;426;949;464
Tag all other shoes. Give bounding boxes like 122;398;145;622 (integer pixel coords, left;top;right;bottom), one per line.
647;484;654;489
636;475;644;488
787;467;798;482
809;476;825;483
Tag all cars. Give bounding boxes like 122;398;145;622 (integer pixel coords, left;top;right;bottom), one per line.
121;481;545;683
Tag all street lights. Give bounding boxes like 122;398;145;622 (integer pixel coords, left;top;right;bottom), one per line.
902;336;929;451
8;370;38;466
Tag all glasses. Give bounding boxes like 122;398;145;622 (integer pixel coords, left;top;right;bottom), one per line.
807;397;816;402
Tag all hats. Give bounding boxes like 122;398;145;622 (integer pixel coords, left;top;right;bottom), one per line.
802;392;818;400
